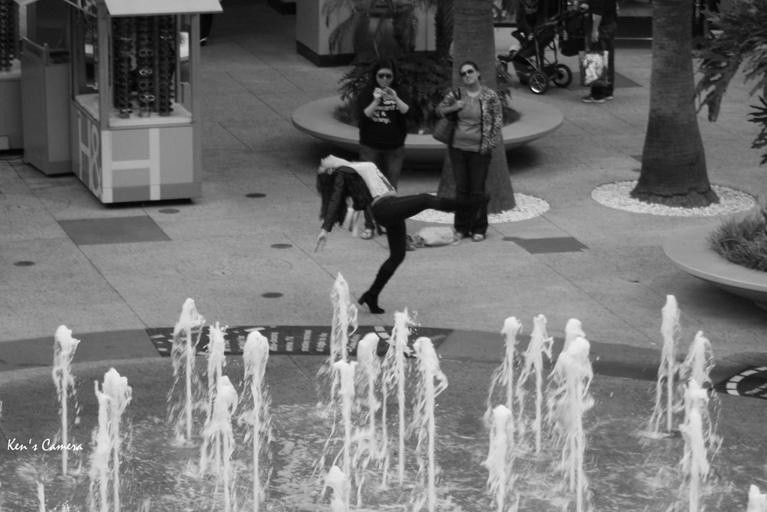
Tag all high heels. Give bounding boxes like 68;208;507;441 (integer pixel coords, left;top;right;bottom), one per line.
358;291;384;313
454;194;480;216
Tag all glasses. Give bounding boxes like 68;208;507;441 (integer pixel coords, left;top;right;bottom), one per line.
461;69;473;77
378;72;391;78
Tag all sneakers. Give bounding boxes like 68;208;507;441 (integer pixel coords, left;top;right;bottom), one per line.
454;231;485;241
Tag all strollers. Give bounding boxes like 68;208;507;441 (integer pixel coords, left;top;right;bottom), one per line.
494;1;578;95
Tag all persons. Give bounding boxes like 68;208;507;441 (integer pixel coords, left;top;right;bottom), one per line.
315;155;490;314
435;62;502;243
581;0;616;103
359;64;412;240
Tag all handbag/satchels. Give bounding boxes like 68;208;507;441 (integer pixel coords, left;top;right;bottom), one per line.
433;118;455;144
